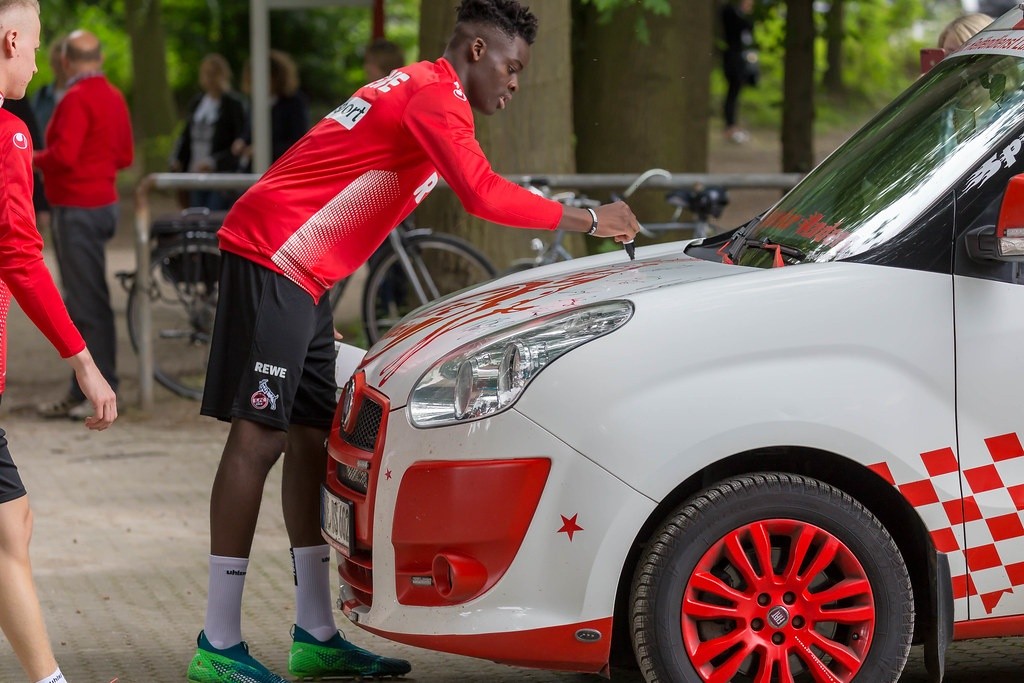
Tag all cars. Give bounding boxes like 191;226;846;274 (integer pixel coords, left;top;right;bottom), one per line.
322;0;1024;682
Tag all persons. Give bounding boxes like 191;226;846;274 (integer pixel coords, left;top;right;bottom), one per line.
2;91;50;227
184;0;642;683
232;51;306;168
33;28;134;418
365;41;404;83
33;42;68;289
170;54;245;302
937;12;1024;163
0;0;117;683
723;0;759;142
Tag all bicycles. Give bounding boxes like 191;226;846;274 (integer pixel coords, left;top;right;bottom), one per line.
507;167;736;271
113;204;498;403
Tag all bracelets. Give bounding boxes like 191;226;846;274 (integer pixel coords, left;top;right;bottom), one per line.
587;208;598;236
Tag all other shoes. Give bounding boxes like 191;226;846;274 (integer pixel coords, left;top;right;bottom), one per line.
725;127;756;147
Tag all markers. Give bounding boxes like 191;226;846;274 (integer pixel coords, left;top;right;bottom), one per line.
613;195;636;261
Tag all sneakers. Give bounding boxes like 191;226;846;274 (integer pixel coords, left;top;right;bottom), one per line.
288;623;412;680
36;397;97;422
187;629;291;683
